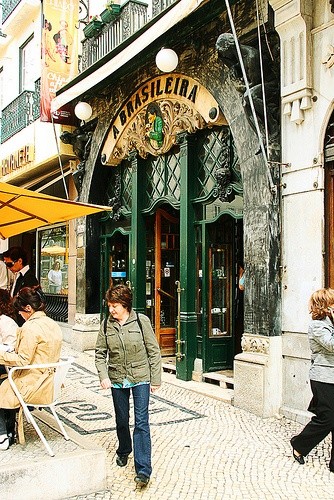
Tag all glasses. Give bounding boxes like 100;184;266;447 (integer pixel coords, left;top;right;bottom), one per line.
4;259;16;268
16;289;31;307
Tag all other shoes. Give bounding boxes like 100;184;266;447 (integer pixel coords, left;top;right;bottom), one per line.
293;447;305;465
116;452;129;467
133;475;150;486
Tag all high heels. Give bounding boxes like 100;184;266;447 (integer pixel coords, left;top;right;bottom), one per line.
6;431;16;444
0;434;9;450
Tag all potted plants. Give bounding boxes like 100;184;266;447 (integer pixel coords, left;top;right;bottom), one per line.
83;0;121;38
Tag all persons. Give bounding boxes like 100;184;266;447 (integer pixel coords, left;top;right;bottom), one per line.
0;246;62;451
291;288;334;473
47;259;63;294
239;267;245;290
94;285;162;486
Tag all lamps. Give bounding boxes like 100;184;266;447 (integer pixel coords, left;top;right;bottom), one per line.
75;93;110;121
155;33;201;73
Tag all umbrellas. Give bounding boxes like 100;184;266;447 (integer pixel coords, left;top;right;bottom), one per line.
0;182;113;240
41;245;69;263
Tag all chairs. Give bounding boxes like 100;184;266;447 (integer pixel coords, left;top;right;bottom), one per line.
9;356;75;457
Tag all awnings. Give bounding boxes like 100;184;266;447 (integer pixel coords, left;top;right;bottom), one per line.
51;0;239;115
